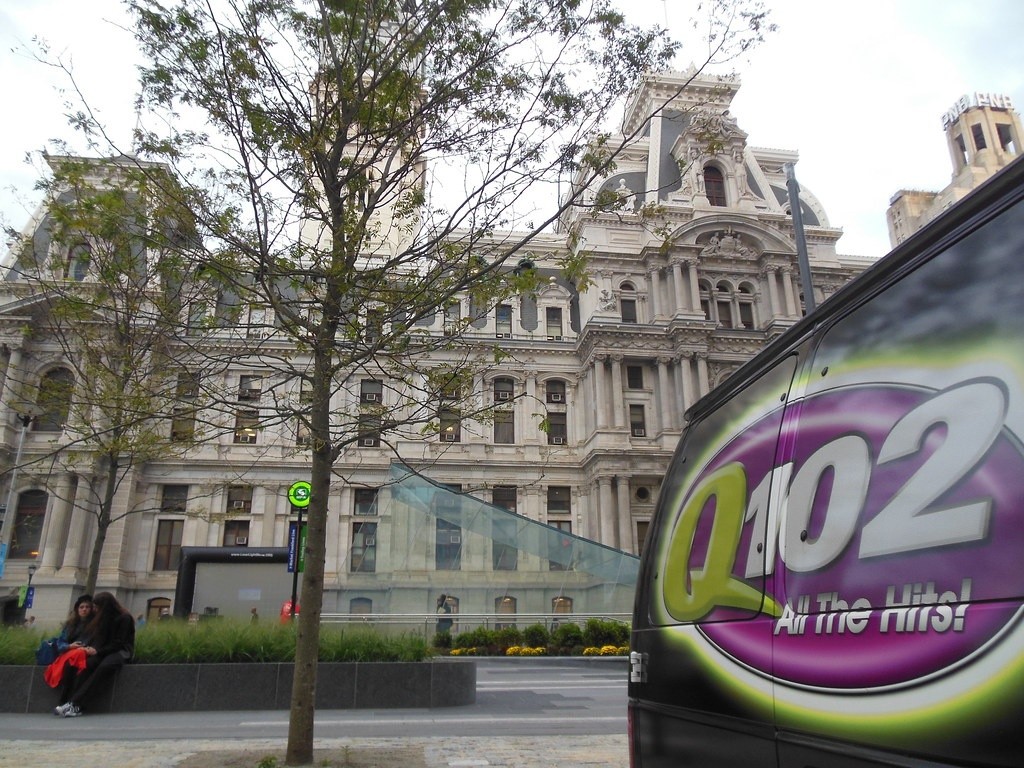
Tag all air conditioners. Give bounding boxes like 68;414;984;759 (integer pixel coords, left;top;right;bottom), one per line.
234;501;243;508
184;389;192;396
635;429;645;436
552;394;561;402
446;434;456;440
367;394;376;401
366;538;375;546
447;391;456;398
253;332;381;343
450;535;459;544
240;436;250;443
450;330;460;336
503;333;512;339
554;335;562;341
553;437;563;445
499;391;509;400
303;437;312;444
364;439;374;446
236;537;247;545
239;389;249;397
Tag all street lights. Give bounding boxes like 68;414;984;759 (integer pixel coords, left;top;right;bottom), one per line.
19;564;36;625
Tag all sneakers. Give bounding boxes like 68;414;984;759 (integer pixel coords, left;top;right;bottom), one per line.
56;699;82;717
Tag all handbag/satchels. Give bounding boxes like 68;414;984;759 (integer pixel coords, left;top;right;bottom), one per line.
35;637;59;665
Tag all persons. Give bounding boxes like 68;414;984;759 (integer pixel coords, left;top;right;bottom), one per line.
709;231;720;254
250;607;259;625
159;609;172;622
55;592;135;717
734;234;749;253
280;593;300;624
720;110;743;131
29;616;36;631
435;594;453;635
